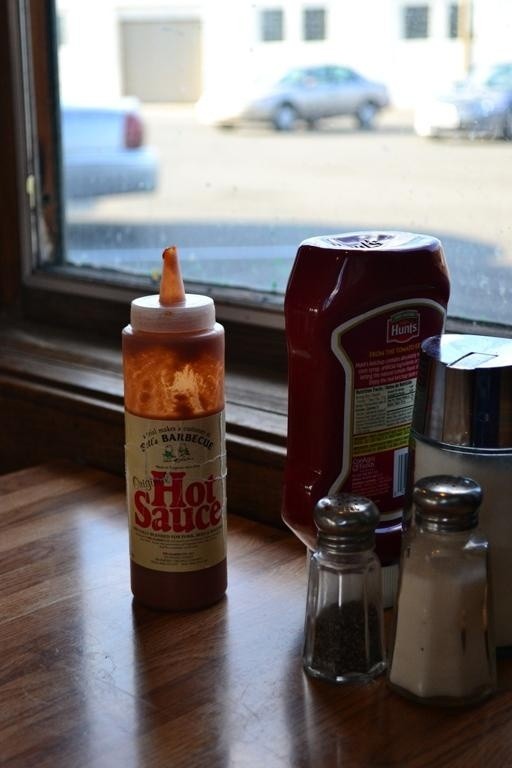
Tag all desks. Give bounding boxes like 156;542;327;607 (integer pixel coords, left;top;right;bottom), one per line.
0;440;511;768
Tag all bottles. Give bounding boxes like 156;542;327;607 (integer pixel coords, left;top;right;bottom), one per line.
406;335;512;651
283;237;451;610
279;487;390;687
116;240;236;612
387;464;502;700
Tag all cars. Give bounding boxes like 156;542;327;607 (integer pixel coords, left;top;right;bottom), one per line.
247;63;393;137
55;95;169;202
412;65;512;144
